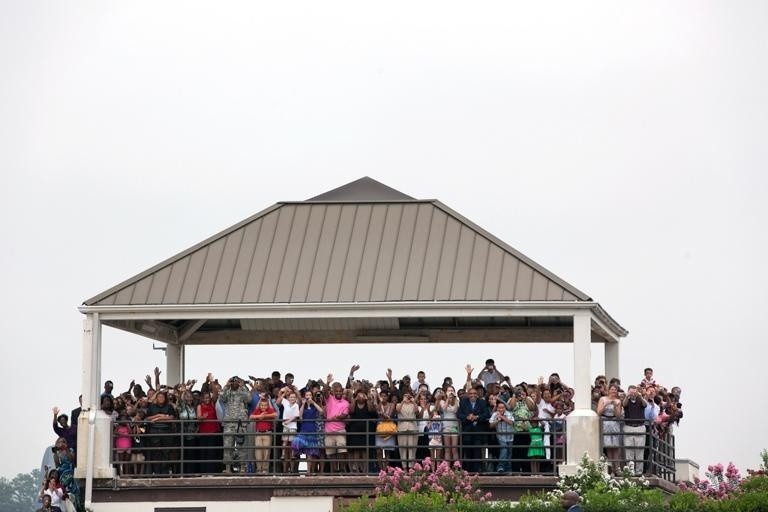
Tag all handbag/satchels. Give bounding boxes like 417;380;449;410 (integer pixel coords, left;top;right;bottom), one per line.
235;419;245;444
375;415;398;440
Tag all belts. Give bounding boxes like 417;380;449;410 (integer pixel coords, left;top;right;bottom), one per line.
626;423;643;427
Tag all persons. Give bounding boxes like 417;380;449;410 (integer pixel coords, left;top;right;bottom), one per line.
38;395;85;512
591;368;683;476
101;359;574;476
